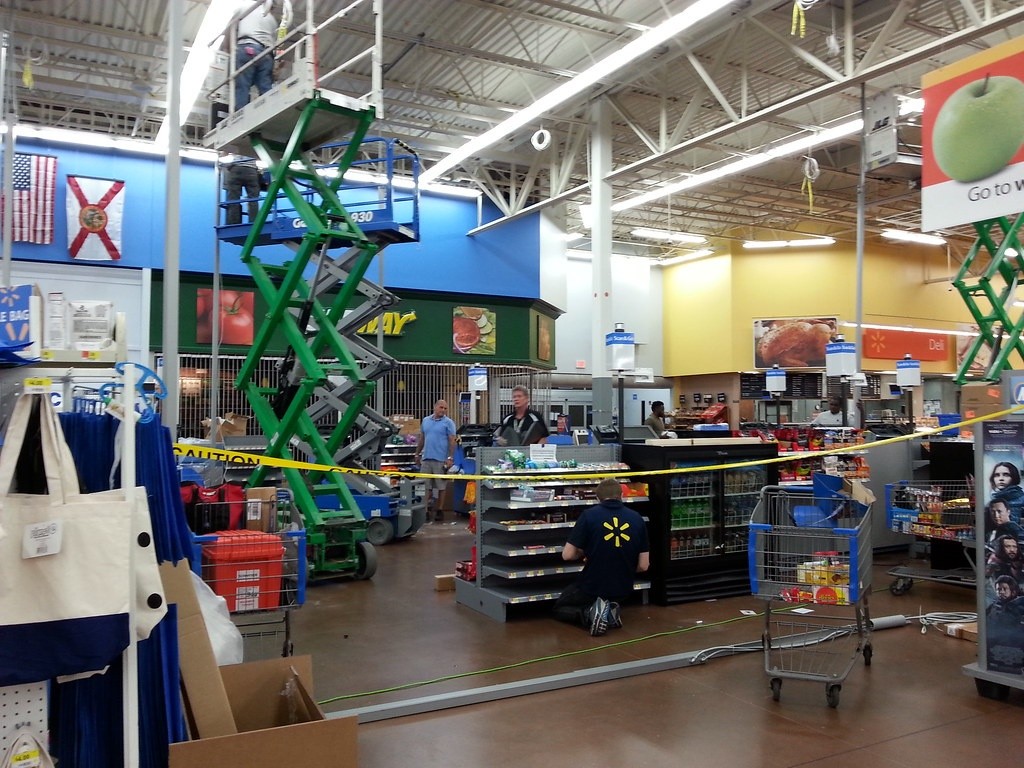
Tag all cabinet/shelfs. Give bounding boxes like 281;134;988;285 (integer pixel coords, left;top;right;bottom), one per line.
455;416;1024;703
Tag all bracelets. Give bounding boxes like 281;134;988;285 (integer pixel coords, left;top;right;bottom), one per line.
415;455;419;458
448;457;454;460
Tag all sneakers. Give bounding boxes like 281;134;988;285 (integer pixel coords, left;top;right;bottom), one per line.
607;602;623;628
589;597;609;636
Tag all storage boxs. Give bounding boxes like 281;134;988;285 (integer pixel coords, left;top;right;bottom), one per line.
167;655;359;768
962;628;978;642
455;562;477;582
434;574;458;591
390;413;421;436
797;565;849;586
943;622;978;642
961;381;1005;433
811;585;851;606
200;529;285;613
201;412;253;442
247;487;277;533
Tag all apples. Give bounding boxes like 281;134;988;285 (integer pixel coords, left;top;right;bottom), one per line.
932;74;1024;182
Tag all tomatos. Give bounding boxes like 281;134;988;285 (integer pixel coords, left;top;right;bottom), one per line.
197;289;254;345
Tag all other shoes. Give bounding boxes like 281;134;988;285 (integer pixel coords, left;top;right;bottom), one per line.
434;510;444;521
425;511;431;521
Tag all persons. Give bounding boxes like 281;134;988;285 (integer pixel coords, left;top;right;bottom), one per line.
217;135;261;224
984;462;1024;676
227;0;279;112
492;385;550;446
415;399;456;522
644;401;669;439
810;396;855;427
548;479;650;636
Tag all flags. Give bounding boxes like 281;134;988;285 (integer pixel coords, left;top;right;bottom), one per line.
0;150;56;245
66;173;127;261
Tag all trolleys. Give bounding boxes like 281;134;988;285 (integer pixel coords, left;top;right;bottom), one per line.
885;476;981;592
745;481;873;708
187;502;307;658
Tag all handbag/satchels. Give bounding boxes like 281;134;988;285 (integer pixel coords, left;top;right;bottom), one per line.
0;384;168;687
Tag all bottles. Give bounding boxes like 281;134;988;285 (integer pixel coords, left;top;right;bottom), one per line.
669;458;766;558
